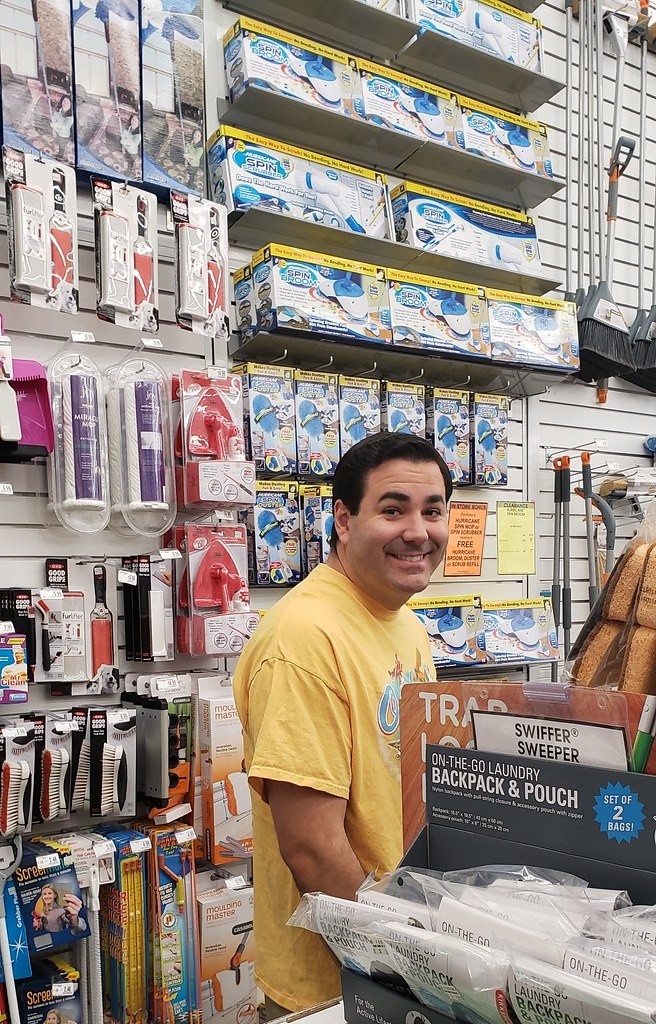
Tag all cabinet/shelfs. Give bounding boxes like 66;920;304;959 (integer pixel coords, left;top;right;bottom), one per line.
213;1;570;400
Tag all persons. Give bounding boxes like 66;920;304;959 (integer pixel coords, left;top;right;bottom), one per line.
44;1008;78;1024
182;127;204;189
49;94;75;160
119;115;142;178
40;885;83;930
230;431;453;1023
